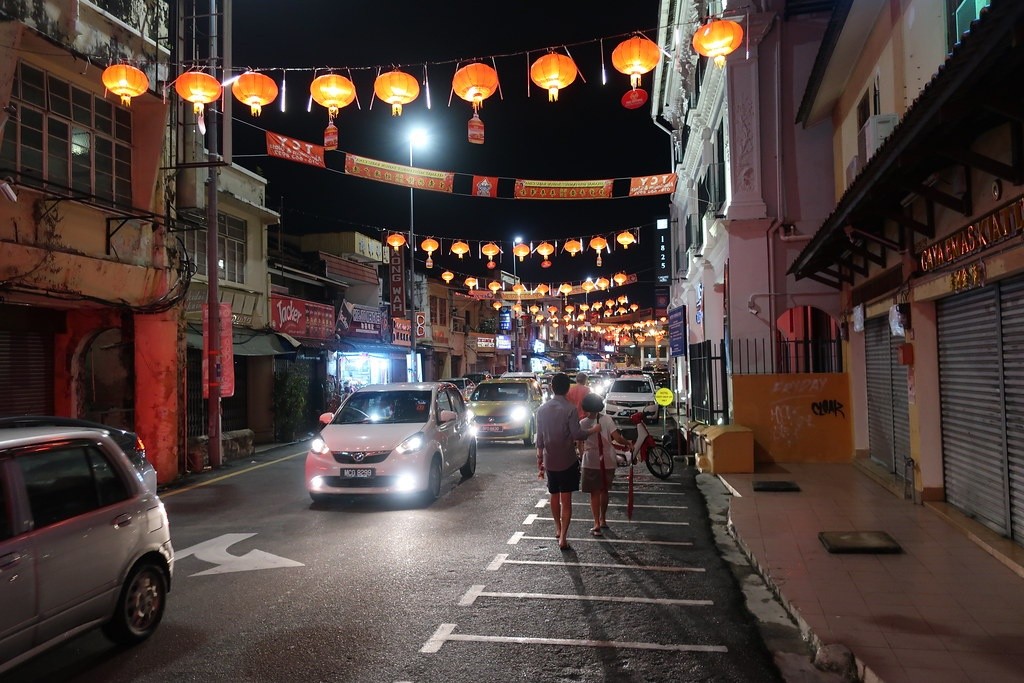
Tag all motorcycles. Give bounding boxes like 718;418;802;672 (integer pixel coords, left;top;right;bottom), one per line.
574;403;673;480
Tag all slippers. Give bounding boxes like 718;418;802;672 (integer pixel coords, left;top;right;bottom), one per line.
560;542;571;550
590;528;601;535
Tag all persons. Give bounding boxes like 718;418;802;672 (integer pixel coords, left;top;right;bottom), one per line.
536;373;601;550
567;373;591;459
576;393;634;536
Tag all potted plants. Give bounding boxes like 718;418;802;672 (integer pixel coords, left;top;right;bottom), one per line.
276;373;310;442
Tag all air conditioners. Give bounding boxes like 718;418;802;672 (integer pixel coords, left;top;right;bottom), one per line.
857;113;897;171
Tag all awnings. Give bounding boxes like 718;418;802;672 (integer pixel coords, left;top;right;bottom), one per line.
537;356;558;367
586;354;604;362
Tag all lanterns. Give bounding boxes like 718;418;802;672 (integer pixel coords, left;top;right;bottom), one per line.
421;239;439;256
175;71;221;114
442;230;666;342
231;72;277;117
310;74;355;117
451;63;498;108
387;234;405;251
612;36;661;89
374;71;419;115
101;62;149;108
530;53;576;101
693;19;743;67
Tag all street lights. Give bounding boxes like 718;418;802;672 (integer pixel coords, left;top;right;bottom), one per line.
408;126;430;379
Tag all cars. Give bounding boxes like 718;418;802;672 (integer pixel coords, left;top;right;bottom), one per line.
464;377;544;448
306;381;479;505
436;371;496;403
0;416;176;677
500;364;667;402
604;377;660;423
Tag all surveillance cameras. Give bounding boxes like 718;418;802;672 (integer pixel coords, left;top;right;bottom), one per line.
751;310;757;315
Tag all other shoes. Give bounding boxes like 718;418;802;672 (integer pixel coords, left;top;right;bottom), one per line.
599;523;609;528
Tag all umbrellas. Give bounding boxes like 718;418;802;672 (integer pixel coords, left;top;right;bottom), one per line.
628;450;634;523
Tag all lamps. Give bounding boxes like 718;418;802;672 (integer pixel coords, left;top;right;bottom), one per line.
656;216;680;229
